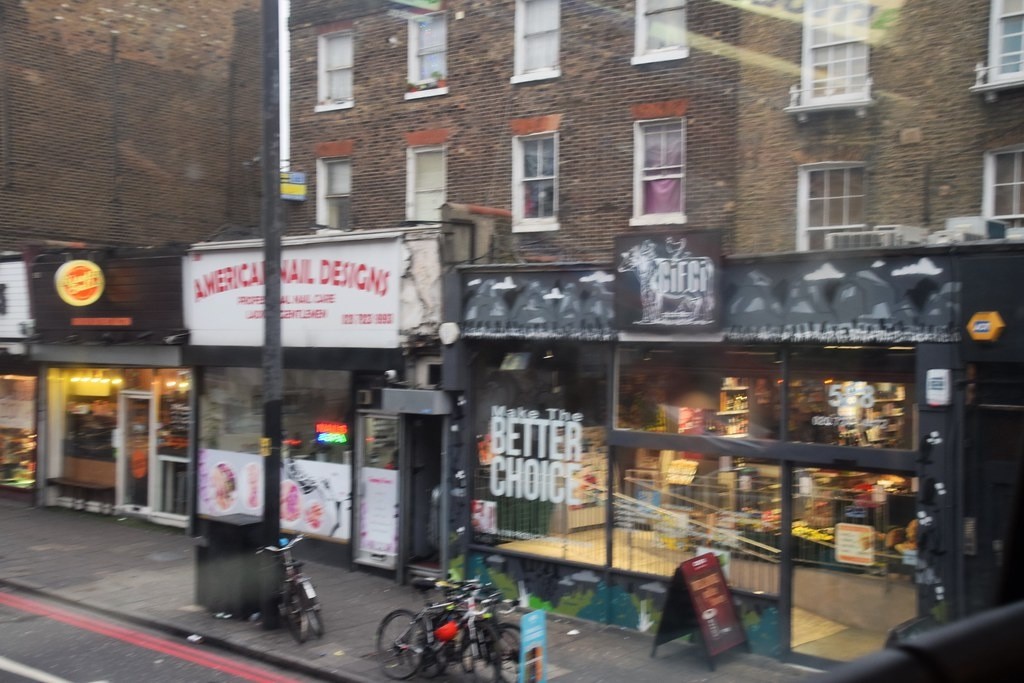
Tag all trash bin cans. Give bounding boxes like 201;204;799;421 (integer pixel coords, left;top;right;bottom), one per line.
196;511;267;620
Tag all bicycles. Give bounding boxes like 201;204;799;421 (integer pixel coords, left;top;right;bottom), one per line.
373;579;523;683
257;532;326;640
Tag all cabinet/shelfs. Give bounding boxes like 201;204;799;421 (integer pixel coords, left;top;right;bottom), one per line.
861;382;904;447
717;385;749;472
